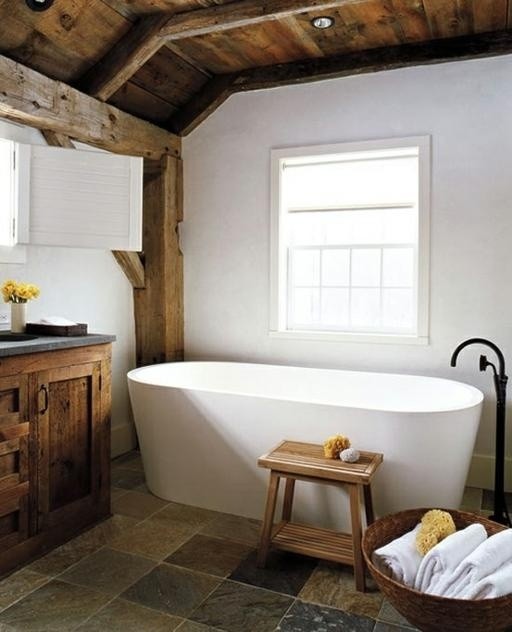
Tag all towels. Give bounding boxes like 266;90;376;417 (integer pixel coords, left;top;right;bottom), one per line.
374;510;512;600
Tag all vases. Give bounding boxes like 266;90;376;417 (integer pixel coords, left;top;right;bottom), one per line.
11;303;27;334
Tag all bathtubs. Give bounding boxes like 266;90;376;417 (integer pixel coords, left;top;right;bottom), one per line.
127;361;484;535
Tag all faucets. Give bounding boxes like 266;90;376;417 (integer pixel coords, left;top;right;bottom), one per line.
450;338;511;376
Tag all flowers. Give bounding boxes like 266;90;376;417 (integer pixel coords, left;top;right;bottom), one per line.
0;279;41;303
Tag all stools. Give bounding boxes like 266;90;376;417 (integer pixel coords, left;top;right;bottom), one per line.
257;439;384;592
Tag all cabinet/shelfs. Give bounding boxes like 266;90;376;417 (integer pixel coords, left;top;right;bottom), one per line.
1;334;117;580
